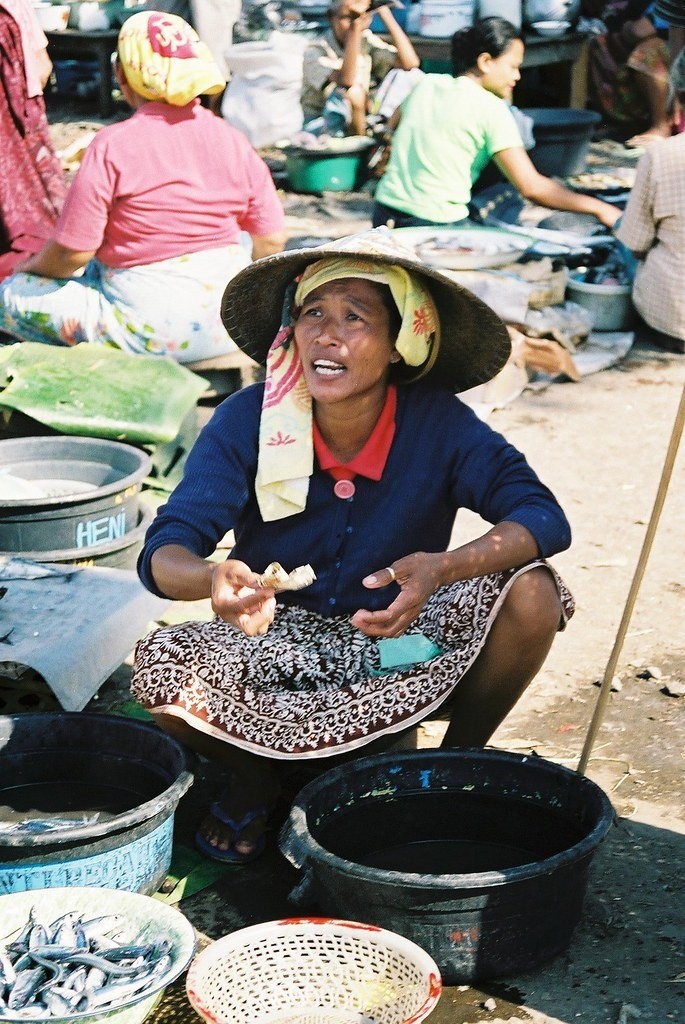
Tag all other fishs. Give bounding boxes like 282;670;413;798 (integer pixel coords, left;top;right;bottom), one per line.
0;905;173;1021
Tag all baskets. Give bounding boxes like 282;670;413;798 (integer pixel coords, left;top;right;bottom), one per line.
185;918;443;1024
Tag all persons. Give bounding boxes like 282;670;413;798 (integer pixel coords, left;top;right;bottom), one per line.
130;225;573;864
0;11;288;366
621;82;685;354
300;0;422;136
620;0;685;145
371;15;626;228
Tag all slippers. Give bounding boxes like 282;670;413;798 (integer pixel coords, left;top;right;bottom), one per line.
194;784;276;865
625;133;665;149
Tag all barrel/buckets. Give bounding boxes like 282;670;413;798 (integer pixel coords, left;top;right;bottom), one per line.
0;436;155;572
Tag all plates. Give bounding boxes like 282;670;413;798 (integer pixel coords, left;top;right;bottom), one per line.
388;227;535;271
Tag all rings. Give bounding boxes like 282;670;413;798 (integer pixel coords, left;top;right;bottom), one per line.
386;567;395;581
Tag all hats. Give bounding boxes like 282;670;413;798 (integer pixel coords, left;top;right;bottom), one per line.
220;225;512;396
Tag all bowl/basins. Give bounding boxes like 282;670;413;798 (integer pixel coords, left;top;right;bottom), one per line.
567;268;633;331
0;712;206;897
275;138;375;196
532;21;571;36
0;886;196;1024
278;747;616;986
520;109;601;178
